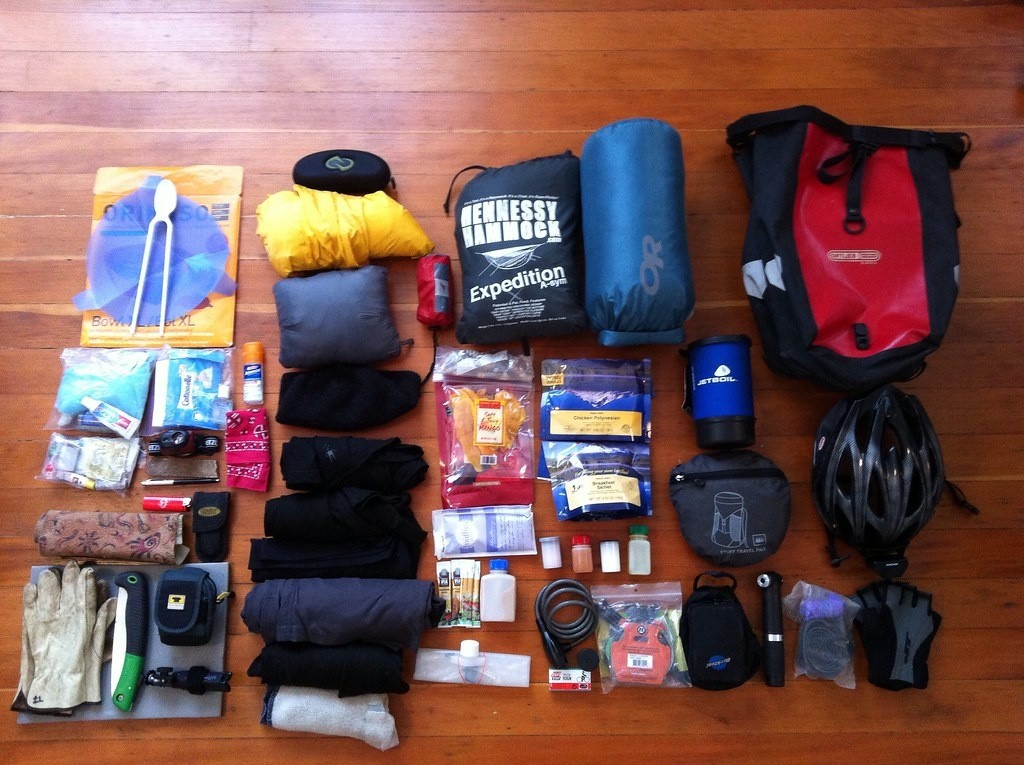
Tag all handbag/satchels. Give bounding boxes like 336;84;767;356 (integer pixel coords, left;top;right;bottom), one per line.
724;106;972;398
679;571;763;692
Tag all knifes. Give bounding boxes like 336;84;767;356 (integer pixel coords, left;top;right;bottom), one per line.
109;571;149;712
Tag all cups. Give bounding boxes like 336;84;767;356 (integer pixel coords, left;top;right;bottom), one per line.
678;333;757;449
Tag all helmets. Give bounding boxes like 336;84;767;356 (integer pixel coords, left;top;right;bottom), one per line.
809;383;983;580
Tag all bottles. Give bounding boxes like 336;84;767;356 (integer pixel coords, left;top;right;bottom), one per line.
242;339;265;405
480;524;653;624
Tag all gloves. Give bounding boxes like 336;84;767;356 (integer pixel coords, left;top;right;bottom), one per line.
9;559;118;717
